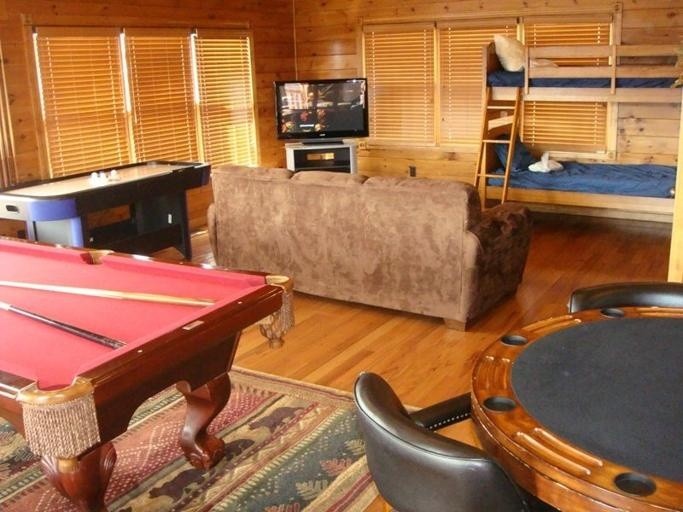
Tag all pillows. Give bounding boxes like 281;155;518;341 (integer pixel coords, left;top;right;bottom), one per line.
493;132;537;174
494;34;527;72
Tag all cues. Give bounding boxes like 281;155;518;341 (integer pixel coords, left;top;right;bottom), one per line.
0;280;213;309
0;300;125;351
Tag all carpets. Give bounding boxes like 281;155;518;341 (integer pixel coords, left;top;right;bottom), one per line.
0;360;420;511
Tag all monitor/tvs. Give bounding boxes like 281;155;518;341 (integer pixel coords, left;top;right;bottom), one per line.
274;77;369;145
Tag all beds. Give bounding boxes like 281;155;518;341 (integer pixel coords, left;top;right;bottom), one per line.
467;41;683;217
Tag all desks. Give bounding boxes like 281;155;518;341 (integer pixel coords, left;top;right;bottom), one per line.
1;162;211;260
1;233;294;510
467;291;683;507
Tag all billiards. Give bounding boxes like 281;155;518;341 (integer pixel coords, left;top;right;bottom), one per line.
88;169;118;179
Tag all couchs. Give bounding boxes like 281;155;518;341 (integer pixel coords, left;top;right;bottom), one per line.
204;160;533;330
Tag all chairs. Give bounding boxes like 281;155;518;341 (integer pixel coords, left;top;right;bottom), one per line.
352;372;546;511
